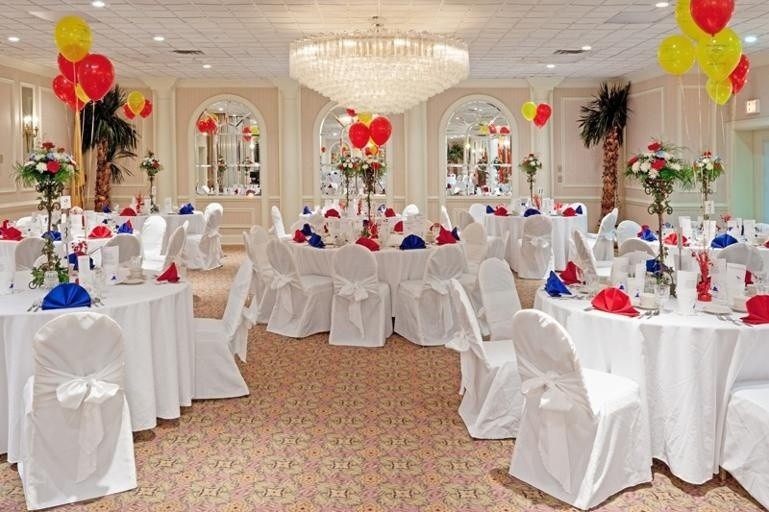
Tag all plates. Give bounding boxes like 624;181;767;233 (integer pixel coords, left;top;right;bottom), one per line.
729;305;749;313
702;304;731;313
114;278;144;285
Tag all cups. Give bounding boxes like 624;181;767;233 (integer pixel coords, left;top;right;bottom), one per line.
43;271;58;289
313;218;431;248
575;262;698;314
747;271;769;297
97;245;147;279
674;217;756;245
734;296;751;308
78;256;91;281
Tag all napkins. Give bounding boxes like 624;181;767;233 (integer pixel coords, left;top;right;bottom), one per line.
157;262;181;283
545;270;572;297
591;287;640;318
40;283;91;310
559;261;586;285
739;294;768;326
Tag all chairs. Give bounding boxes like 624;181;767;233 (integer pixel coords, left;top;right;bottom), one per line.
456;238;504;338
265;238;334;339
244;233;278;327
2;201;224;280
250;200;768;280
449;277;527;442
393;243;470;347
508;308;653;510
19;309;139;511
479;255;522;343
718;379;768;511
327;244;396;347
191;259;258;400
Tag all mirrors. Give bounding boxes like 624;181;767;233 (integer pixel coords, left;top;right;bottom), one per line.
442;101;513;196
193;99;260;196
320;104;388;197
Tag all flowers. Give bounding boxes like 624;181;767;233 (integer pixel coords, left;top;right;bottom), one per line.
337;154;387;218
213;153;252;185
139;151;163;202
14;142;83;286
619;142;726;282
516;152;542;198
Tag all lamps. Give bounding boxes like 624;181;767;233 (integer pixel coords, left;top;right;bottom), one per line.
287;15;469;115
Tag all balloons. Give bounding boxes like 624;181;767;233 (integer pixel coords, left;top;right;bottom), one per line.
242;127;260;140
197;112;219;135
365;145;381;169
657;0;750;105
534;115;547;127
521;101;537;121
346;107;391;148
481;124;509;135
537;103;552;118
52;14;115;112
121;91;152;121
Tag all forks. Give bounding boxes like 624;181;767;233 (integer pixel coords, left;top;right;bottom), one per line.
713;313;740;326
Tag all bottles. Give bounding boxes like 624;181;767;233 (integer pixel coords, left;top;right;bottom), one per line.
66;263;79;285
